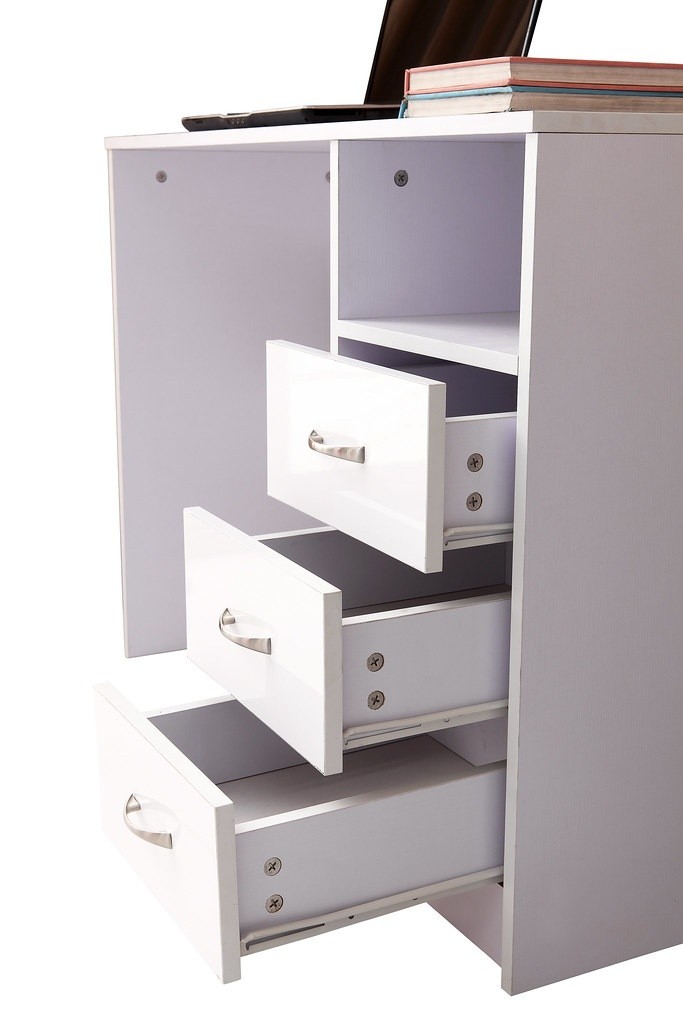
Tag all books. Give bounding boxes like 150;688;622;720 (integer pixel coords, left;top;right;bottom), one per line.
398;57;683;120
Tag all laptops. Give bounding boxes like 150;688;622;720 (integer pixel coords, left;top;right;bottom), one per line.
181;0;541;132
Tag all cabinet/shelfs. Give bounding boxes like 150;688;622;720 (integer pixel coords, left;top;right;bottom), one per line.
101;109;683;997
